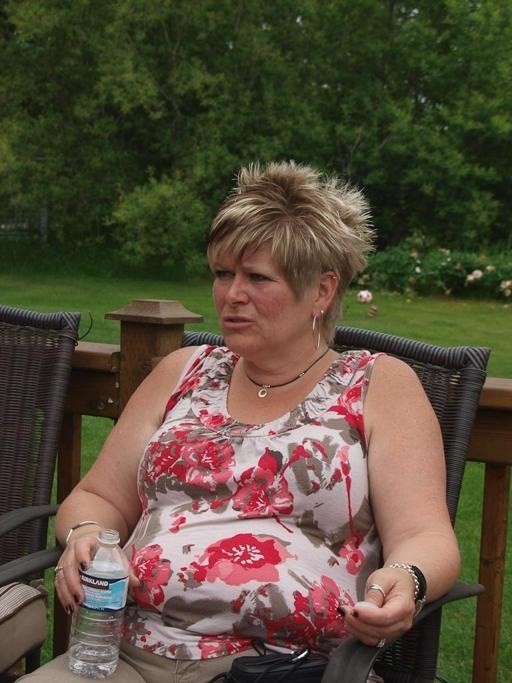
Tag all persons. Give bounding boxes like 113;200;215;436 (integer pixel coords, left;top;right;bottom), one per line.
13;155;465;682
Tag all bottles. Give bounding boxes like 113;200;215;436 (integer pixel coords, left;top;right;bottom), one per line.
67;529;131;680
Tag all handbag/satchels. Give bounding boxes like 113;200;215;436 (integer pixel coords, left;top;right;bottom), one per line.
228;650;329;683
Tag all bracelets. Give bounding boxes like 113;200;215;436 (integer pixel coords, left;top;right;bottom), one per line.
65;520;100;545
385;562;428;618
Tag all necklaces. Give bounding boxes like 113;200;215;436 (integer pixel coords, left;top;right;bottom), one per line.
245;346;330;400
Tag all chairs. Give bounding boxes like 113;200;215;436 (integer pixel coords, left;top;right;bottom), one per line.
0;305;93;682
0;324;492;683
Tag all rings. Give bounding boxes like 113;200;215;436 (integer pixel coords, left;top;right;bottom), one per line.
53;566;63;573
366;584;386;600
376;638;387;648
